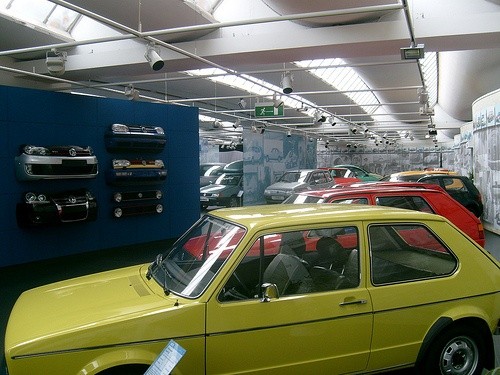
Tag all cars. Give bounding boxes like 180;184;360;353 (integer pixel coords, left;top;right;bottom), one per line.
264;168;337;203
408;174;484;221
319;164;457;189
4;202;500;375
277;186;486;259
198;158;243;191
199;172;245;208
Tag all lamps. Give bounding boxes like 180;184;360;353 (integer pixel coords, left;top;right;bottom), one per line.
144;43;165;72
46;51;67;76
280;72;294;94
213;89;438;150
125;85;139;100
399;42;424;60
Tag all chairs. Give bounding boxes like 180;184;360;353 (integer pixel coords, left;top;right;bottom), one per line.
242;232;310;298
296;236;353;294
